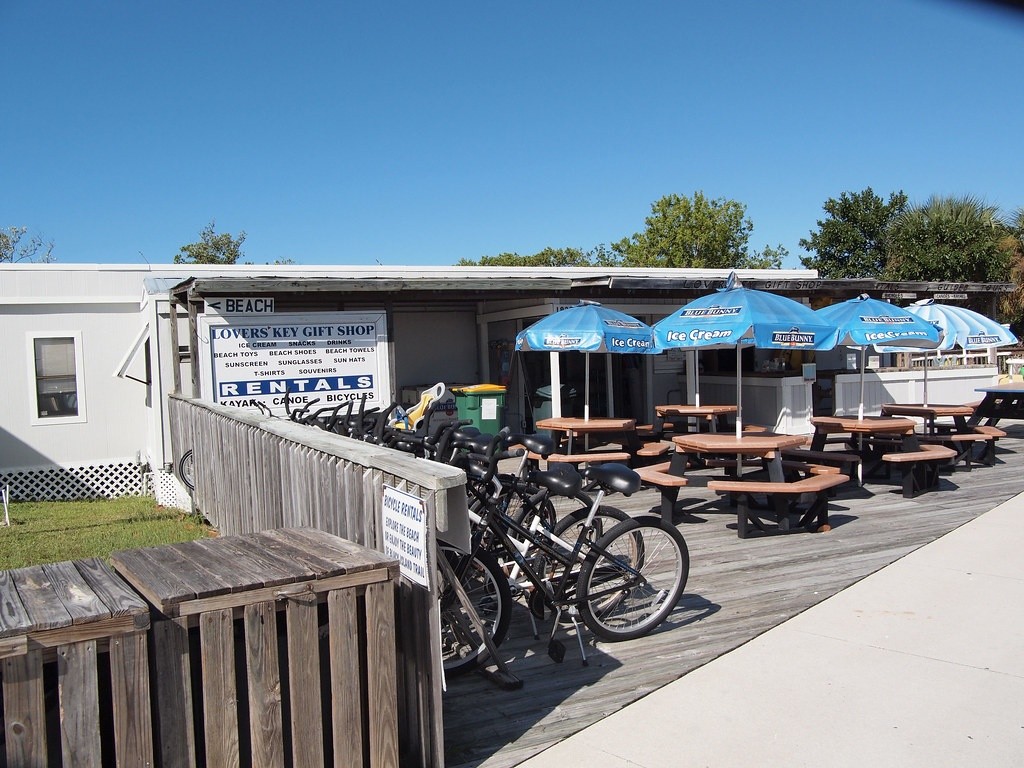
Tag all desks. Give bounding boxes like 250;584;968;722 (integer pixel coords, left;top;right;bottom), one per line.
810;415;917;480
966;389;1024;426
881;403;975;459
655;404;737;434
536;417;637;455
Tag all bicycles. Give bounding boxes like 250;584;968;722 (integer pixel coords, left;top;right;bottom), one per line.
178;382;690;690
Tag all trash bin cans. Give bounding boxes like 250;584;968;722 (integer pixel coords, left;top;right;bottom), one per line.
532;383;577;441
448;384;506;436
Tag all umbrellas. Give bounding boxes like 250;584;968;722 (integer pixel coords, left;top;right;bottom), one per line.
814;294;940;487
847;299;1018;434
653;271;840;477
515;300;662;486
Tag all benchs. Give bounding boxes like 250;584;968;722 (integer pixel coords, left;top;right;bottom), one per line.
547;452;631;467
743;423;768;432
782;449;860;486
636;423;674;436
882;445;957;498
926;423;1007;466
706;473;849;539
508;439;569;475
873;433;993;472
637;443;670;465
704;459;806;507
773;461;841;497
631;461;690;524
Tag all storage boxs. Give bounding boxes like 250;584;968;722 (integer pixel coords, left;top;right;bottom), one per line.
401;383;473;437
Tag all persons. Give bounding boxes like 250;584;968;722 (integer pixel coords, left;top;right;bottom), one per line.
785;350;816;370
